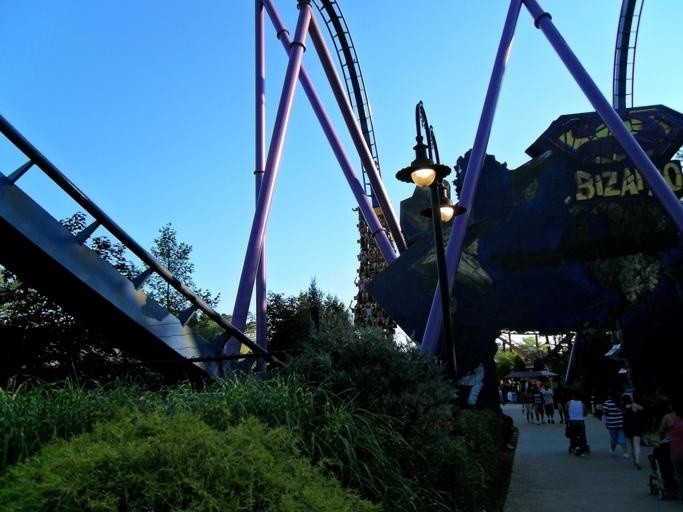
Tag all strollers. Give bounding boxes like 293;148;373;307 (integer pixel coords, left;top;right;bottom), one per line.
564;418;590;454
646;437;678;500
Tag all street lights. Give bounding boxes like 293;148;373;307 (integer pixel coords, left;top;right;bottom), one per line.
395;99;467;387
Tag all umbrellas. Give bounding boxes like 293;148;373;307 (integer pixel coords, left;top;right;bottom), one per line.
505;371;532;378
530;371;559;377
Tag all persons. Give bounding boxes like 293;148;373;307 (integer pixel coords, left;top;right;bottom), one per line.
564;391;589;458
623;395;646;471
660;402;683;479
500;379;569;425
601;392;625;451
